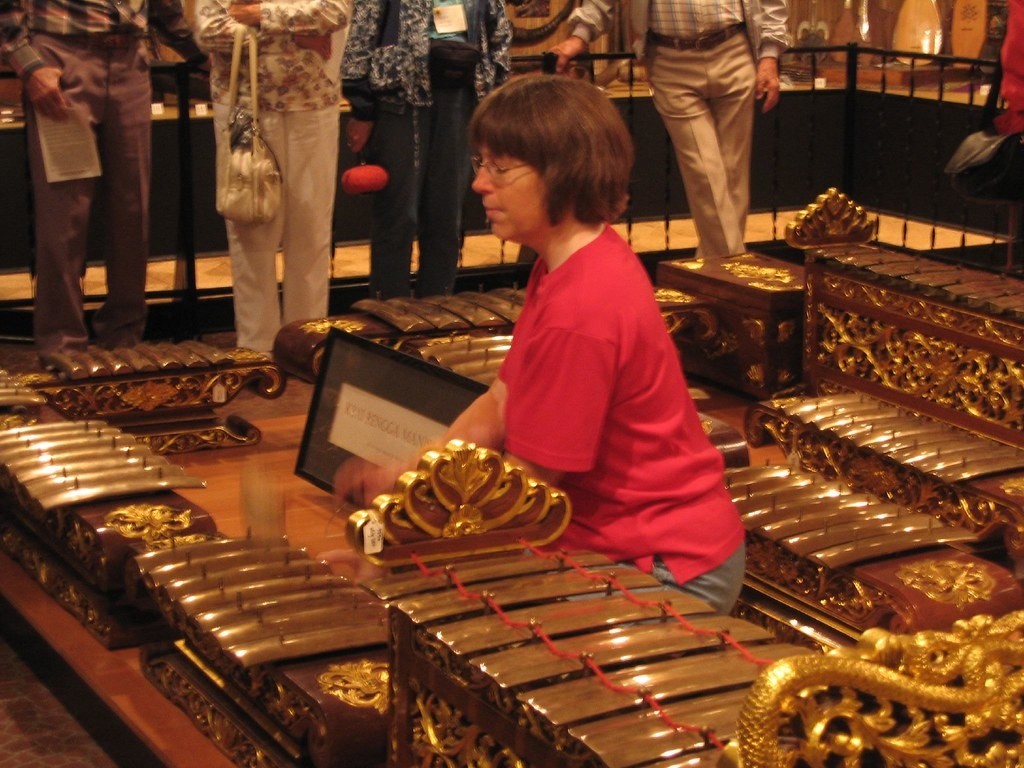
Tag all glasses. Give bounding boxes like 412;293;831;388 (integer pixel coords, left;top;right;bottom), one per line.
471;155;532;180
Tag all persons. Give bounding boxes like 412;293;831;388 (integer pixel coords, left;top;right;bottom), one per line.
337;0;513;302
178;0;355;365
537;0;791;258
331;73;747;619
0;0;212;355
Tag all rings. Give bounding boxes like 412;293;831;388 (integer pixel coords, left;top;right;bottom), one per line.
346;143;352;148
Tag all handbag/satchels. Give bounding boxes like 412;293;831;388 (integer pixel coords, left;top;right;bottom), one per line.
214;31;282;224
943;129;1024;202
429;39;482;94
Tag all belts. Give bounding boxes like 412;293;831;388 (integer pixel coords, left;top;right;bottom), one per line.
64;34;141;49
652;23;746;51
